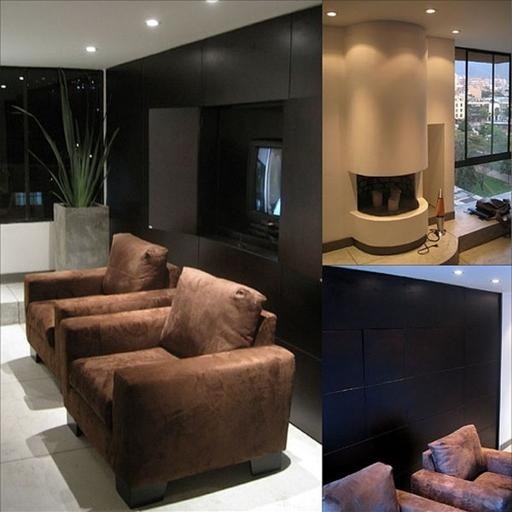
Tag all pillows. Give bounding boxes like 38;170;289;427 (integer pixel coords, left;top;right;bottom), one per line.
426;423;488;481
159;267;267;358
103;232;168;295
323;461;400;512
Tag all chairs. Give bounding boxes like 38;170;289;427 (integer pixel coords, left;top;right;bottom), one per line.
24;261;180;393
323;489;466;512
412;449;512;511
58;307;296;510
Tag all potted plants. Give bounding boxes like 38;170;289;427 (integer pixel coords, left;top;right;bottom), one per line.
13;68;110;272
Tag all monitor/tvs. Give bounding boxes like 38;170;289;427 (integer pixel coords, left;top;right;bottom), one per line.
246;138;282;241
